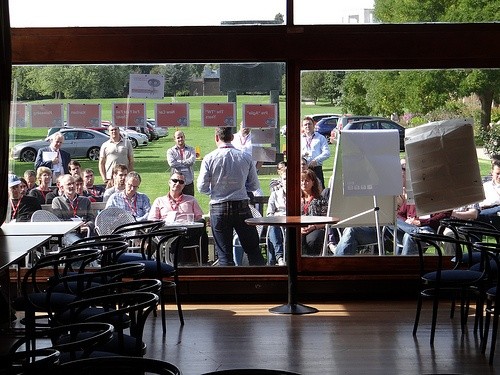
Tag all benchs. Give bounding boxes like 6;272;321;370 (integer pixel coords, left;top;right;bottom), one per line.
207;196;270;261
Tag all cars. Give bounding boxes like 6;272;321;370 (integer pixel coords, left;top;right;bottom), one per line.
11;128;111;163
46;116;169;149
280;113;407;151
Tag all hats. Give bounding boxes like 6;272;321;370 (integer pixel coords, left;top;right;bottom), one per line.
8;174;21;187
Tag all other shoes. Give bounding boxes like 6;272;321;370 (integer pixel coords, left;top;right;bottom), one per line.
419;230;434;234
278;258;285;266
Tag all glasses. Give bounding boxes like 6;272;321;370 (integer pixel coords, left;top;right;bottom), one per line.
170;179;185;184
494;171;500;175
128;183;139;189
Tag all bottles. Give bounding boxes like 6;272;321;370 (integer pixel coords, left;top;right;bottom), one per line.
196;146;200;160
283;142;286;157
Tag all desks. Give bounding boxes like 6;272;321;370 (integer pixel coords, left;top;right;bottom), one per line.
1;221;86;266
0;235;52;296
245;216;339;315
162;221;204;265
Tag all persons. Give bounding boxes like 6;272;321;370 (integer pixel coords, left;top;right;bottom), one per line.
167;130;195;196
231;120;264;172
146;172;209;265
196;127;268;267
300;117;331;190
34;132;71;186
98;124;135;183
264;158;500;266
9;159;151;268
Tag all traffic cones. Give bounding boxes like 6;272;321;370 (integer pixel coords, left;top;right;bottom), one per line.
194;146;203;160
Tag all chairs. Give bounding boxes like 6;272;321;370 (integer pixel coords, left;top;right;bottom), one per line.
409;217;500;369
6;219;186;375
94;207;141;253
31;210;60;222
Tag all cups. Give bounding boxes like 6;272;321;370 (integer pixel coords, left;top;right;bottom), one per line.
187;214;194;224
